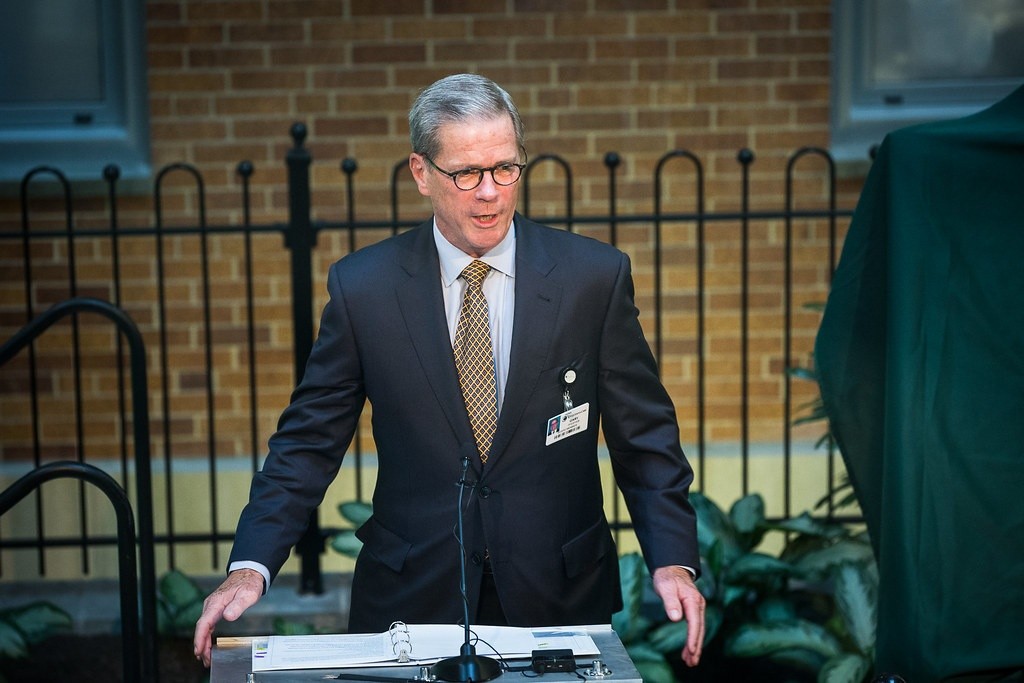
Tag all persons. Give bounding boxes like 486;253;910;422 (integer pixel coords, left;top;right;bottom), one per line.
548;419;559;436
194;73;706;668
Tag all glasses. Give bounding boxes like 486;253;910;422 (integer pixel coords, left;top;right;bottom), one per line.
420;144;527;191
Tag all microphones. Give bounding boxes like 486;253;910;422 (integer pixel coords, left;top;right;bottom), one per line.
427;442;503;682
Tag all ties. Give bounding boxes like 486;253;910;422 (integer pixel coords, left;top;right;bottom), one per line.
453;260;497;466
